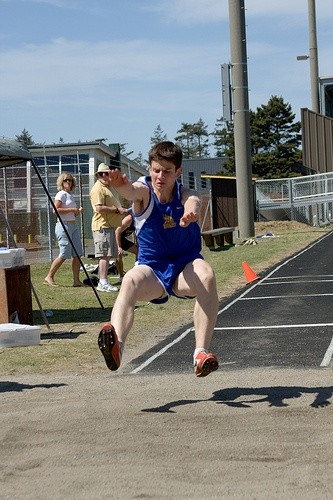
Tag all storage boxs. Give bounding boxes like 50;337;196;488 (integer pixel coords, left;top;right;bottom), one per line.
0;323;42;348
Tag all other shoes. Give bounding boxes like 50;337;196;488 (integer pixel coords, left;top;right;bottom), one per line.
119;276;123;283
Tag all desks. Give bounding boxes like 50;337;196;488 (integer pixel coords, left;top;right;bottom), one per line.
0;264;34;327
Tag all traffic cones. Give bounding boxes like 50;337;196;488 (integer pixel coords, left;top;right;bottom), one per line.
243;260;260;284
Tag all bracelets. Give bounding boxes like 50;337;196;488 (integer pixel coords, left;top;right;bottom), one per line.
115;207;119;214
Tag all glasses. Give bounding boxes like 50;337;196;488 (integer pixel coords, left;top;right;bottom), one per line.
98;172;109;177
63;179;73;182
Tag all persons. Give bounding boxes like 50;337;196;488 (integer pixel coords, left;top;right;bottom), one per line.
45;172;88;287
89;162;126;292
114;211;138;283
97;141;219;378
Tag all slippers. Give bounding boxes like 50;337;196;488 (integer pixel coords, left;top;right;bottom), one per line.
73;282;89;287
43;280;60;287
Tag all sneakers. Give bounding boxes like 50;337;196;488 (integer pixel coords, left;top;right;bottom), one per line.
96;280;119;293
193;351;219;377
97;323;124;371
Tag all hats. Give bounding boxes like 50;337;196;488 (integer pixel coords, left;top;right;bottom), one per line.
94;162;112;178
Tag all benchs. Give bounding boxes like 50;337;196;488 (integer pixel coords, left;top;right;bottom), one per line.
200;226;235;252
